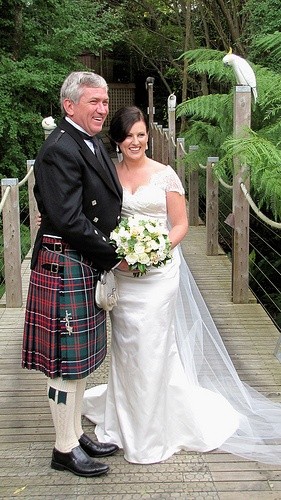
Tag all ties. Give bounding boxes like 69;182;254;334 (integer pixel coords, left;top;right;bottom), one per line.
78;130;115;183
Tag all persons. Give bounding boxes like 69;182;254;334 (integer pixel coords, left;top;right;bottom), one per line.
35;106;241;467
22;71;148;478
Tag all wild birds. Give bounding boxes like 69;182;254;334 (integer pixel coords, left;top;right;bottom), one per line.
222;46;258;105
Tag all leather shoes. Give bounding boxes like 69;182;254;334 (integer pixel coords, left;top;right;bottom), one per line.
76;431;119;458
50;445;110;478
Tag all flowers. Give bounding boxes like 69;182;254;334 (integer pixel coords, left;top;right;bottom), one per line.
108;214;174;277
